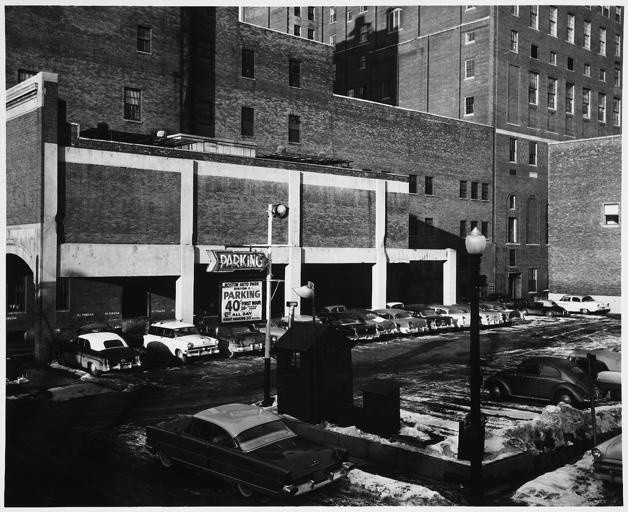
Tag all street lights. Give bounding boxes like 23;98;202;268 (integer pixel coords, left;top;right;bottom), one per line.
458;227;486;460
297;281;321;425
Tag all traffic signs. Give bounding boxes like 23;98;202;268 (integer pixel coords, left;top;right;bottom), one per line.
205;250;271;273
